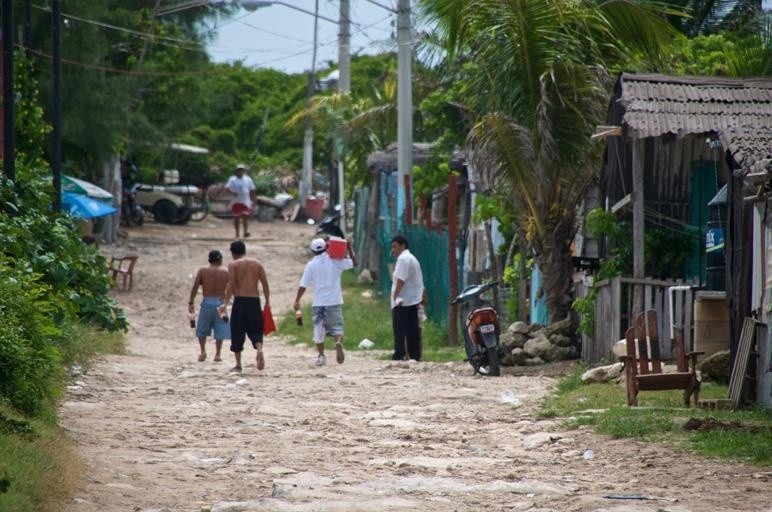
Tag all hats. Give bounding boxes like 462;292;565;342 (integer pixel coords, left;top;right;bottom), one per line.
310;237;326;252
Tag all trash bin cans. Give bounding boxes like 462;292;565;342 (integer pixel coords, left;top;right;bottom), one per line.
306;195;325;221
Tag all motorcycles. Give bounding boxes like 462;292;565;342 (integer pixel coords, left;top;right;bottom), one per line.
306;205;358;270
450;279;504;376
122;178;146;226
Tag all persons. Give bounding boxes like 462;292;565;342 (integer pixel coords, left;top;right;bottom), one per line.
291;236;359;367
225;164;258;240
385;235;425;364
217;240;272;374
187;250;232;363
411;285;430;356
122;178;139;227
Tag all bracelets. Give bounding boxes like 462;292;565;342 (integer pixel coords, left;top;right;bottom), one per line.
188;302;194;305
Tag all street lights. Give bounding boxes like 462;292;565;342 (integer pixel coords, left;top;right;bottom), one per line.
240;0;354;104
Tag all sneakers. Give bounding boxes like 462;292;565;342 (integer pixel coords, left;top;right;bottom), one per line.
335;342;345;364
316;354;326;366
257;351;264;371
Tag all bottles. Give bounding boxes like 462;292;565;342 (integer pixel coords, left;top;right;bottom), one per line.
216;307;229;323
296;309;303;326
417;303;425;322
188;308;196;330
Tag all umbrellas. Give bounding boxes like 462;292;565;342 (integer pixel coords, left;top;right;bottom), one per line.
44;192;120;222
36;175;114;202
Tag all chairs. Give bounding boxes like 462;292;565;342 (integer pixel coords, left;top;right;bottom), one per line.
82;236;140;291
159;170;180;192
619;309;704;411
